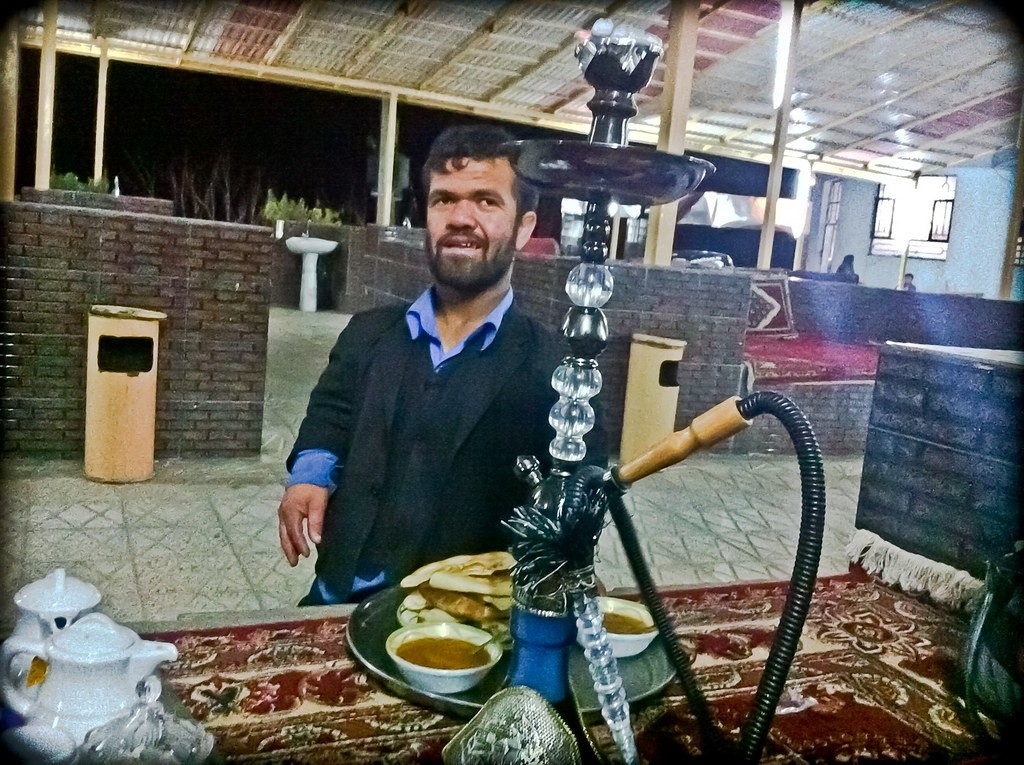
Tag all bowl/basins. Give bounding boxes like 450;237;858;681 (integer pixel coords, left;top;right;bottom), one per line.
385;623;503;694
595;597;658;658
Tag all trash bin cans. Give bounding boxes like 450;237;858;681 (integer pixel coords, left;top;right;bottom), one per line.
619;333;688;472
83;304;168;484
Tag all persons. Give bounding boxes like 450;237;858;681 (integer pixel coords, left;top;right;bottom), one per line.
837;255;854;274
278;126;609;607
903;273;916;291
401;190;420;228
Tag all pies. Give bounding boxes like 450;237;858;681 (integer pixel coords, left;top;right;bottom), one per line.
397;552;516;651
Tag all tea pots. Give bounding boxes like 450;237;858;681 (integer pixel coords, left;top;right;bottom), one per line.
10;568;103;690
0;613;179;747
